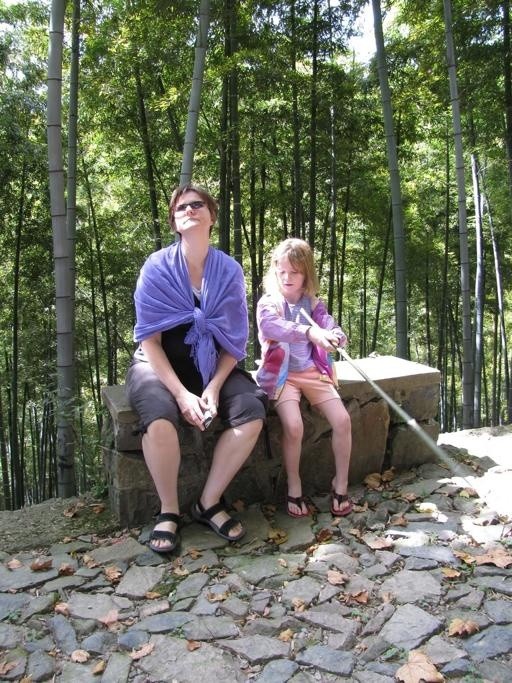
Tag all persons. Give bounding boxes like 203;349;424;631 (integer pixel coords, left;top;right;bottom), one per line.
127;183;266;554
256;239;352;518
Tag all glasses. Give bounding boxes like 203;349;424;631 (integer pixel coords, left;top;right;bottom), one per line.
172;201;209;211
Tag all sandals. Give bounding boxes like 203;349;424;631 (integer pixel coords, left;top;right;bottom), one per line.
191;496;246;541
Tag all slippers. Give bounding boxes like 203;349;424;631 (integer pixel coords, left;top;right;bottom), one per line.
286;490;310;518
330;482;353;517
149;513;183;552
